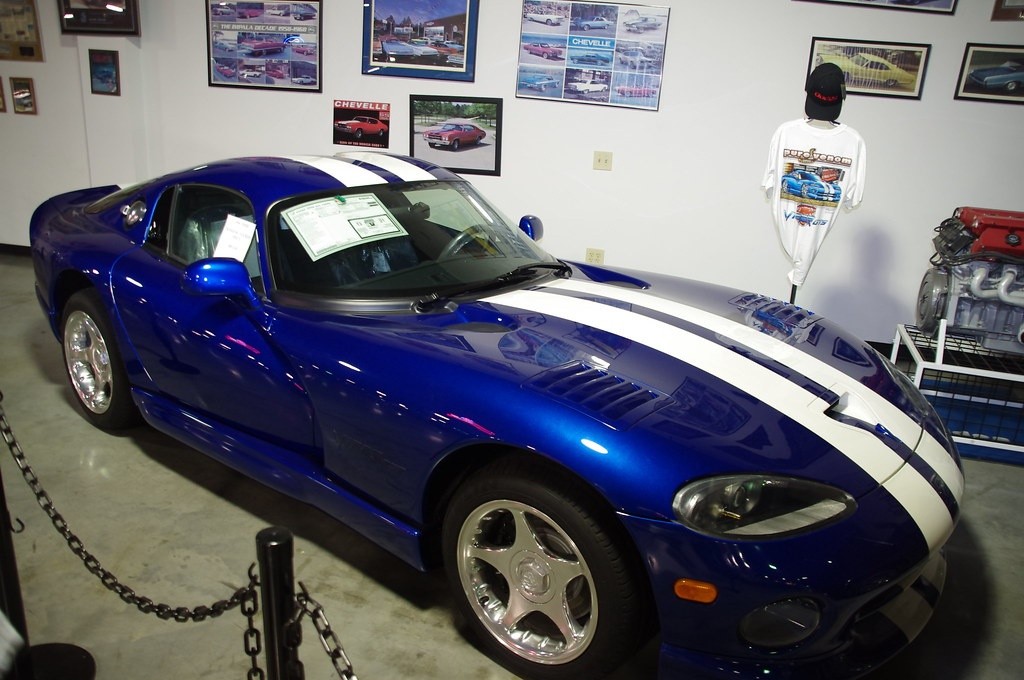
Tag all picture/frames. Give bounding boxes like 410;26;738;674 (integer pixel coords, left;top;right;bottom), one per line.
9;77;37;114
409;94;503;176
0;0;45;63
205;0;324;93
88;50;120;96
362;0;480;82
791;0;1024;106
56;0;141;36
514;0;671;112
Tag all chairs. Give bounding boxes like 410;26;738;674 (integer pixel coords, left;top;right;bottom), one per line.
178;201;264;292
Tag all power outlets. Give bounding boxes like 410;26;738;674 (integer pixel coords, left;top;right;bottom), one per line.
586;248;604;265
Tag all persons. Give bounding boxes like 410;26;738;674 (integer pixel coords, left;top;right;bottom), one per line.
330;201;430;285
763;63;867;293
745;297;815;347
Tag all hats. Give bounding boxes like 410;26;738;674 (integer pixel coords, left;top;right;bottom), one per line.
804;62;846;121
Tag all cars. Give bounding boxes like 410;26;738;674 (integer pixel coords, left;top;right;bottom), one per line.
26;150;966;680
569;53;610;66
624;16;663;33
213;3;317;57
422;122;486;153
523;42;563;58
817;53;916;87
619;42;662;70
380;37;464;63
521;75;560;92
616;83;656;97
240;69;262;78
526;9;565;26
267;69;284;79
568;79;608;94
336;116;388;140
218;64;235;78
574;16;615;31
293;75;316;86
970;62;1024;93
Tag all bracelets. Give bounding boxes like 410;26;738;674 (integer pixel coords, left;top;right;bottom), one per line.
406;205;411;214
